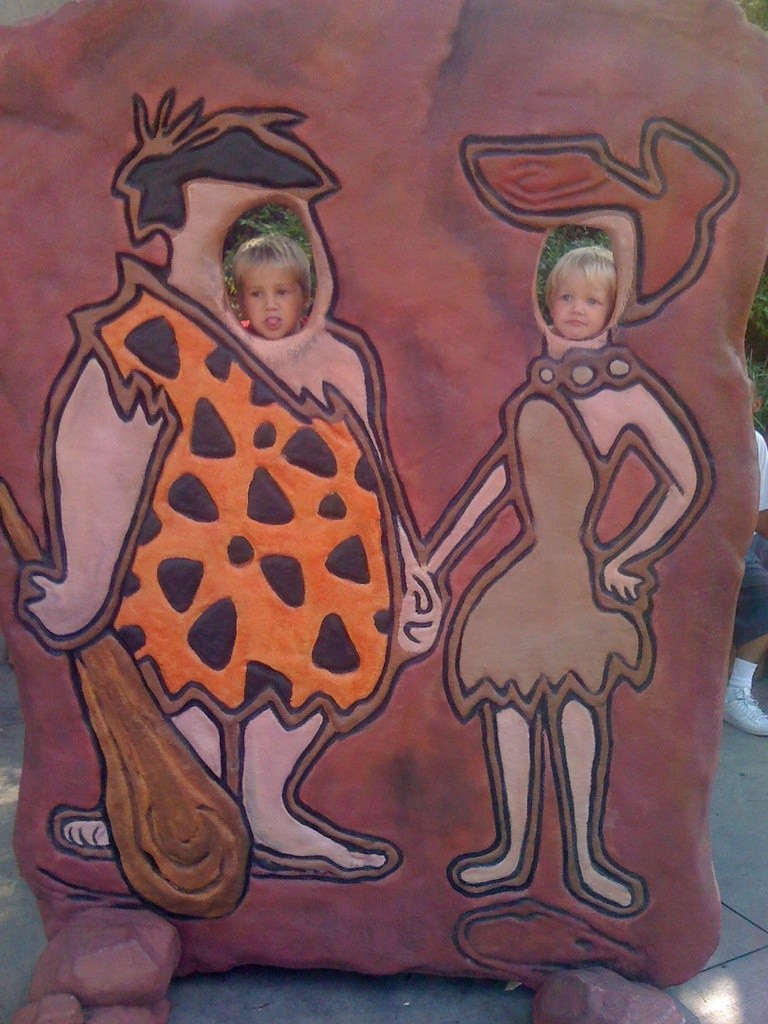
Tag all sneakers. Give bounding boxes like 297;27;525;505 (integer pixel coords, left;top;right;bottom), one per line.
723;684;768;735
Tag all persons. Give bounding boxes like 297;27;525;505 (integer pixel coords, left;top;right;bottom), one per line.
721;381;768;737
232;234;312;340
545;246;618;342
